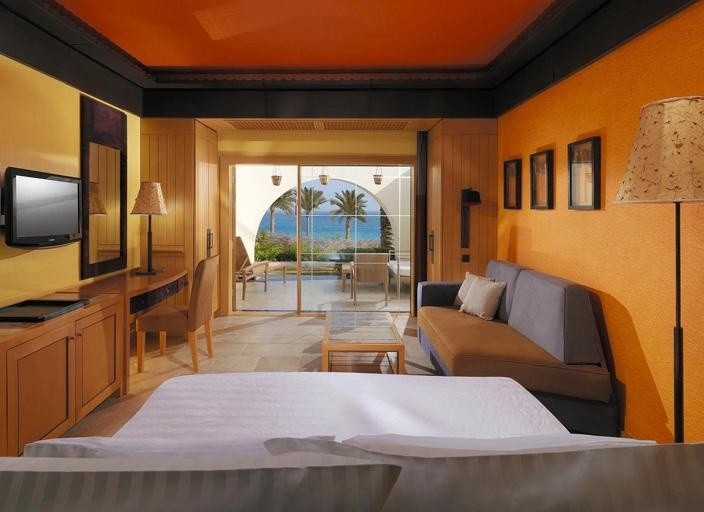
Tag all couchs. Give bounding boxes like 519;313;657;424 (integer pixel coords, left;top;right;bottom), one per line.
414;256;622;437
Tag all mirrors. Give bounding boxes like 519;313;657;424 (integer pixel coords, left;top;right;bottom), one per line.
78;90;128;281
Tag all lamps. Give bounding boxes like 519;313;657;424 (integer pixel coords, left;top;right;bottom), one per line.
128;180;169;276
458;186;481;249
612;95;704;443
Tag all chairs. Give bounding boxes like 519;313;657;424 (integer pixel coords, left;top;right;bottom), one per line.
134;251;222;375
389;254;410;299
349;252;389;308
234;235;269;301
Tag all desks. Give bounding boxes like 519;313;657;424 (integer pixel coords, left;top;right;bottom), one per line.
56;266;189;376
340;264;350;292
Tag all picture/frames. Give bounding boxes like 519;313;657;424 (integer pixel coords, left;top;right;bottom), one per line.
528;148;553;212
565;135;600;212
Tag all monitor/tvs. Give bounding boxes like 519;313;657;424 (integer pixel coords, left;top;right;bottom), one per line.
0;167;82;249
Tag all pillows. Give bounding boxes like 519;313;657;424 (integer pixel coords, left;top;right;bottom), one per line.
452;271;495;308
458;275;507;321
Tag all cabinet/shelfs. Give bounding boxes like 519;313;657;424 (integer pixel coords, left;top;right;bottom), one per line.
0;291;126;456
140;119;220;343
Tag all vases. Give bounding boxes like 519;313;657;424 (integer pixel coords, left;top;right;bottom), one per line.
318;174;329;185
271;175;282;186
372;174;382;184
503;156;521;210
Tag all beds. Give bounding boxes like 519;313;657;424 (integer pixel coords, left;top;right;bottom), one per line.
0;369;704;510
